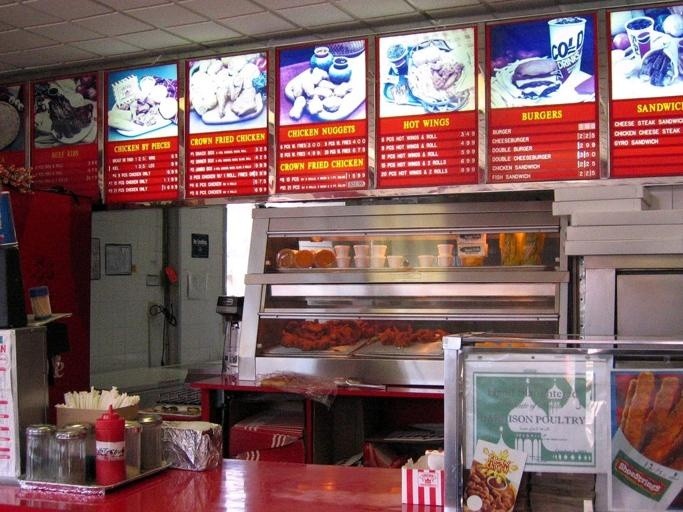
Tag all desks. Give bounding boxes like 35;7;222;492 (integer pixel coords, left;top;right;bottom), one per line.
1;458;450;512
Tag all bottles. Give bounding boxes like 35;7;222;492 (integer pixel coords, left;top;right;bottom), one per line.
306;46;353;85
23;403;164;486
28;285;53;322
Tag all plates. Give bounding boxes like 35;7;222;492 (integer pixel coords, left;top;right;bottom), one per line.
111;103;174;137
0;99;20;152
200;91;264;125
50;118;94;144
285;67;367;122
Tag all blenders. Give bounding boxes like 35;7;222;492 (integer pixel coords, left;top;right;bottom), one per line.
214;293;244;377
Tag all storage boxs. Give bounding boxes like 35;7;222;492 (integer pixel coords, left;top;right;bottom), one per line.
514;472;596;512
56;404;139;457
229;406;305;464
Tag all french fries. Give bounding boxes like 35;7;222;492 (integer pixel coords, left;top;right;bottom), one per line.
620;373;683;471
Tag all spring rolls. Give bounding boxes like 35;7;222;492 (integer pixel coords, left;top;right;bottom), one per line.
636;38;680;89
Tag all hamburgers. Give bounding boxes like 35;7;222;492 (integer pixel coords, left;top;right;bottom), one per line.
509;59;565;103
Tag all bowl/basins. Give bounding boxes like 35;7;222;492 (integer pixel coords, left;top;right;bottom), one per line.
486;475;509;491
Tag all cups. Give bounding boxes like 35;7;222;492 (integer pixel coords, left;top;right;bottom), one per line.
677;39;683;81
624;15;655;63
498;231;544;265
387;44;411;75
547;17;587;88
331;243;454;269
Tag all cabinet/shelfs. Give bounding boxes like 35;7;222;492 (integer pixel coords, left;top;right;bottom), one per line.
237;200;571;388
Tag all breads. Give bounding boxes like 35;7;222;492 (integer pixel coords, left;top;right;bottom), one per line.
274;248;334;268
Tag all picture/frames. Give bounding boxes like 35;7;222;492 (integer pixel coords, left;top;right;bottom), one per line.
90;238;101;280
191;233;209;258
105;243;132;275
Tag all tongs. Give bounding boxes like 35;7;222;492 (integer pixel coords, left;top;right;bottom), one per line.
332;376;388;390
